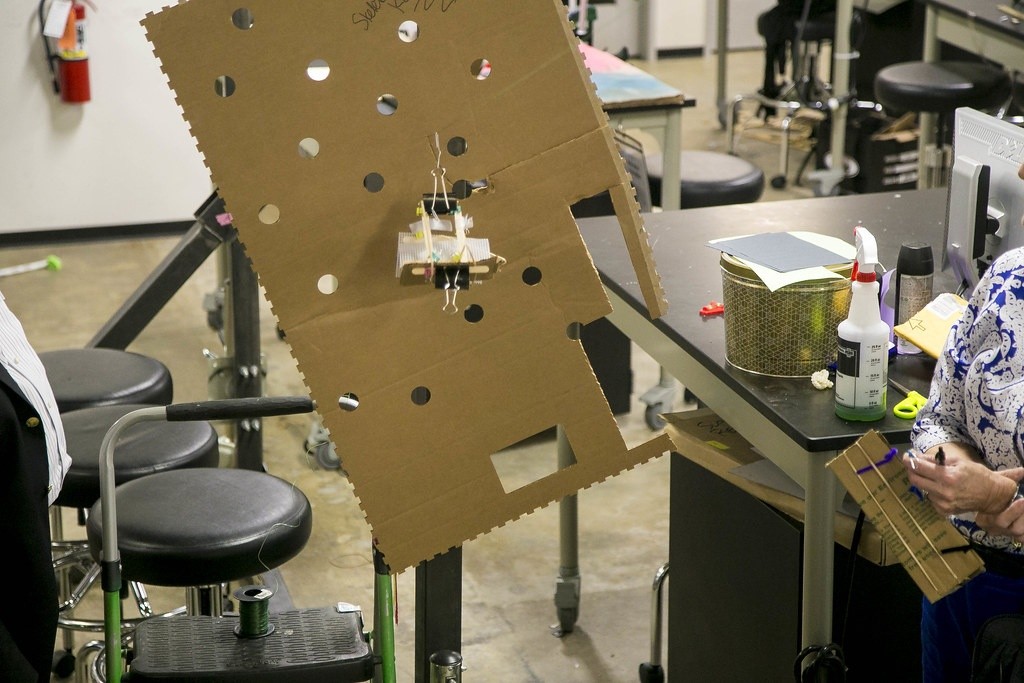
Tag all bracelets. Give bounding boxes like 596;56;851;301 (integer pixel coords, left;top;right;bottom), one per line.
1011;484;1020;505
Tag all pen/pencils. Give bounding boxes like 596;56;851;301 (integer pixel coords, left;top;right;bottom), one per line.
934;446;946;467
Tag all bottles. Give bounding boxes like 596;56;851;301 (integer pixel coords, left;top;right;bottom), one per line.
76;6;86;45
893;241;934;354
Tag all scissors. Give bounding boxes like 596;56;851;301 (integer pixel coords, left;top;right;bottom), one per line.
888;377;928;419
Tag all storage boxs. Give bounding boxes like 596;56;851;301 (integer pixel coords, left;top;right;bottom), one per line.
657;407;901;567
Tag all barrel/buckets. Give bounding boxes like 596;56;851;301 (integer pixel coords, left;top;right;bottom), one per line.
719;250;852;378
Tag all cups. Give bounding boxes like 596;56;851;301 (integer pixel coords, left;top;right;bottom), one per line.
57;55;90;102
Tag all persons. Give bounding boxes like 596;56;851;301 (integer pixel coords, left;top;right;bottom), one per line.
0;292;73;683
901;163;1024;683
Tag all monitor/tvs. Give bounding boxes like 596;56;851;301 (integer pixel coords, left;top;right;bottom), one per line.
942;106;1024;301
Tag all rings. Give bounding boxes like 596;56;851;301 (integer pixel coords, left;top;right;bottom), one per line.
923;491;928;502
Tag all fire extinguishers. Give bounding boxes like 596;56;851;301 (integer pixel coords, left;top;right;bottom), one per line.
38;1;92;104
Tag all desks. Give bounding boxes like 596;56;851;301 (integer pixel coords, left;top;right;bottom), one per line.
300;22;697;211
917;0;1024;189
553;188;973;683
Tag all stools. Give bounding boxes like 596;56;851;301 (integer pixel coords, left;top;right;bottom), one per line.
758;12;835;111
874;62;1012;189
48;404;221;683
646;148;765;211
86;467;312;683
38;347;174;680
